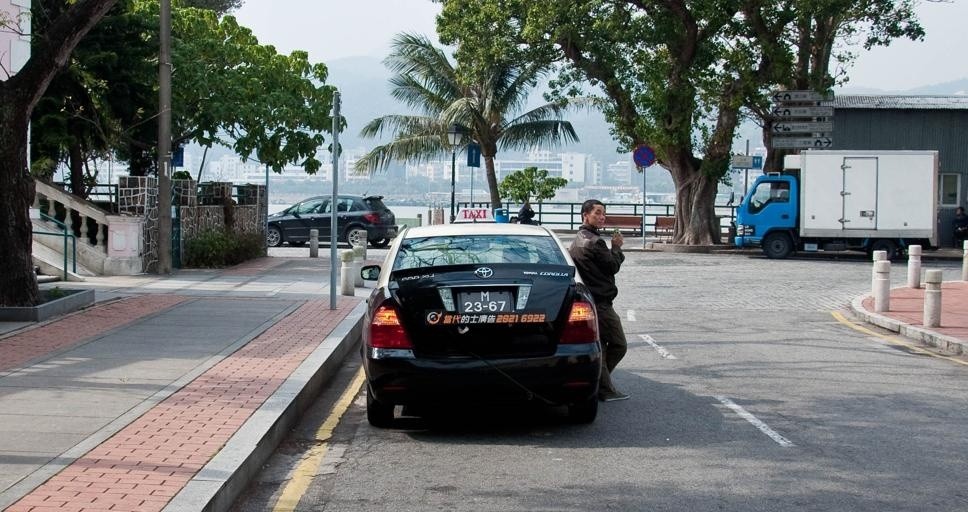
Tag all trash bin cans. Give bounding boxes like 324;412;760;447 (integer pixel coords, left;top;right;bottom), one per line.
495;208;509;243
729;222;736;243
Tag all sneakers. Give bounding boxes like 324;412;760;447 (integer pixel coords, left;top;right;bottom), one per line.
605;391;629;402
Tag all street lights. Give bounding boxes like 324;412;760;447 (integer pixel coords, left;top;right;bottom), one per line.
445;122;463;224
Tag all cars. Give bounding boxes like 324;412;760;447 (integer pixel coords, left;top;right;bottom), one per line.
267;191;398;249
359;207;602;426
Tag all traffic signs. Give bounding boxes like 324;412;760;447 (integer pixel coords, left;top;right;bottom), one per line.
771;105;832;117
771;90;833;102
771;136;832;149
731;155;762;169
771;121;832;134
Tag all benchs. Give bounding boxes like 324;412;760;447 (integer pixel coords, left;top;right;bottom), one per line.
654;216;676;238
597;214;643;237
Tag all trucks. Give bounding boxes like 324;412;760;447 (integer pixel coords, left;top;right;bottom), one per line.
732;148;939;264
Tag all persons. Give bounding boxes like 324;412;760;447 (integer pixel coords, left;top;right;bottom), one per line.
951;206;967;249
517;200;538;226
567;198;631;402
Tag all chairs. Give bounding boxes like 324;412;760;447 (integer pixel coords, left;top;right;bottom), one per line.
780;191;789;198
500;246;531;264
